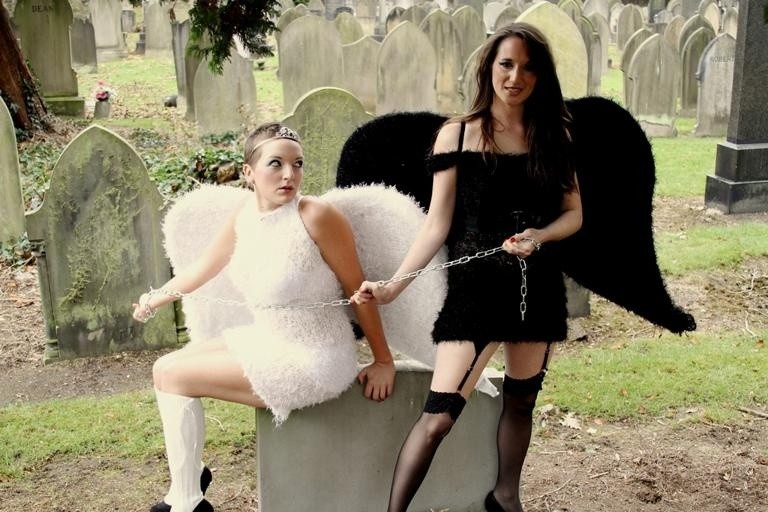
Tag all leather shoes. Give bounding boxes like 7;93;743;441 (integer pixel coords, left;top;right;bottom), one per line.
151;465;214;512
484;490;506;512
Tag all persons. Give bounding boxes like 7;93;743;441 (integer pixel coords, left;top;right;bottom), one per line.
349;23;583;512
130;122;397;512
